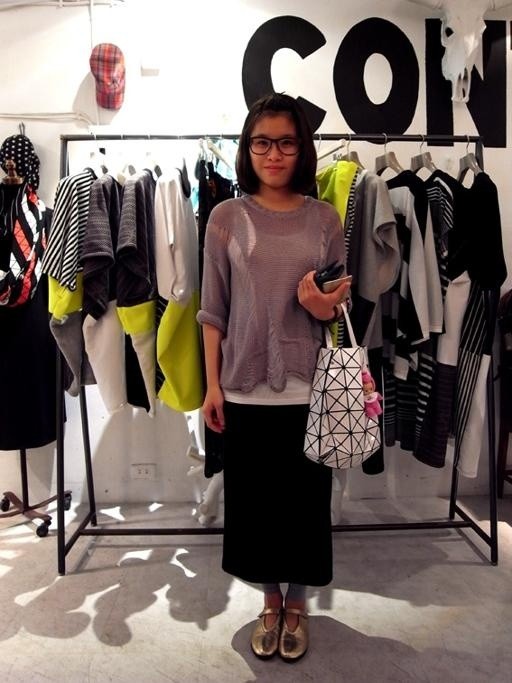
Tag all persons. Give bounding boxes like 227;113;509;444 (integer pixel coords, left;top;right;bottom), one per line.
194;92;354;665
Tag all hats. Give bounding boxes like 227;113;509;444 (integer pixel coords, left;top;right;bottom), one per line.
0;134;40;191
90;43;125;109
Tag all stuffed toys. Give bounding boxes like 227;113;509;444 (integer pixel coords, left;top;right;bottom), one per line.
360;371;384;418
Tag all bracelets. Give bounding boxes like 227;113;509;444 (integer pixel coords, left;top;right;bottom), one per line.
316;306;340;326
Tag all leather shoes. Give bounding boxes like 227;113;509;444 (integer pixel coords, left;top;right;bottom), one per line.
250;608;281;660
278;607;310;664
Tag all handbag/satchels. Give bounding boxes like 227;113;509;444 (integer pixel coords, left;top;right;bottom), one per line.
303;345;382;469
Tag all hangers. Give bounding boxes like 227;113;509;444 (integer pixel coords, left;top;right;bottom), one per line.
65;132;493;195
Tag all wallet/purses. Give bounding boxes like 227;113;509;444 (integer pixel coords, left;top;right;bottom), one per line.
314;260;344;289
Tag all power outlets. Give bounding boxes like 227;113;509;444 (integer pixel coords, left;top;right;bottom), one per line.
130;463;157;479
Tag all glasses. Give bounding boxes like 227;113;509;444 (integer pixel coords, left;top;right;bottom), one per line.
247;136;302;156
